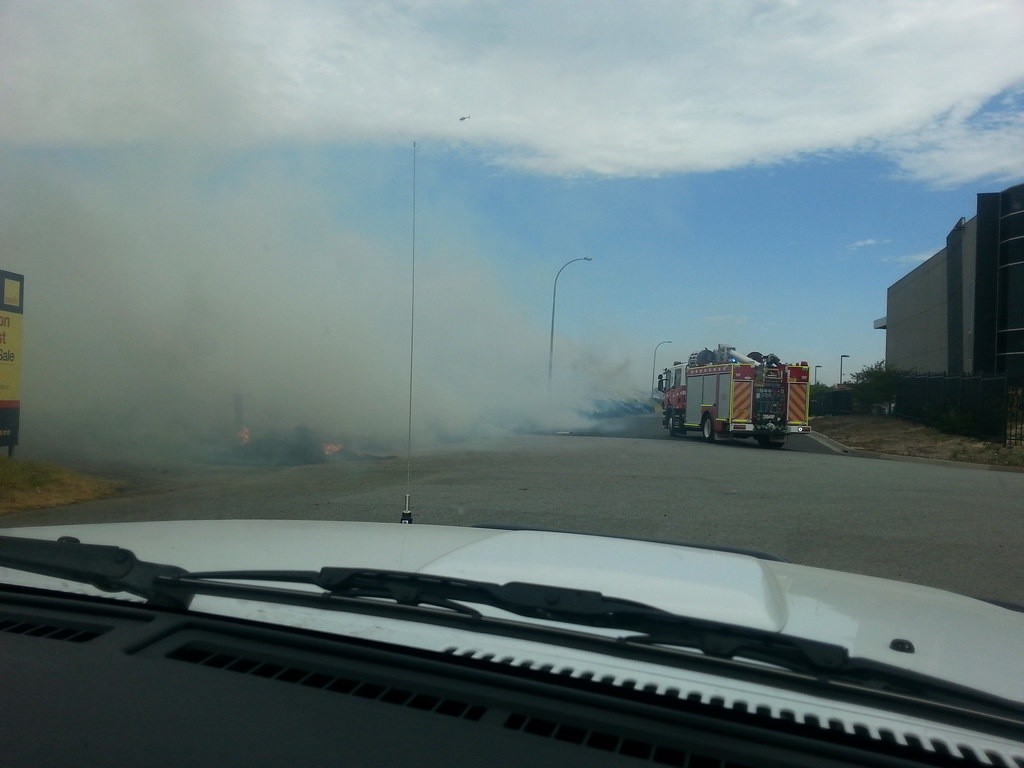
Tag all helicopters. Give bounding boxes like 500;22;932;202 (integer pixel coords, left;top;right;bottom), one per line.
459;116;469;120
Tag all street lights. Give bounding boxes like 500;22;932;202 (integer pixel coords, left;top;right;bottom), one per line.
815;366;822;384
652;341;673;396
547;257;592;384
840;355;849;384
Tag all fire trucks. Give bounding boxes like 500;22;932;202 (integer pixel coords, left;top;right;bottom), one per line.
657;343;812;447
